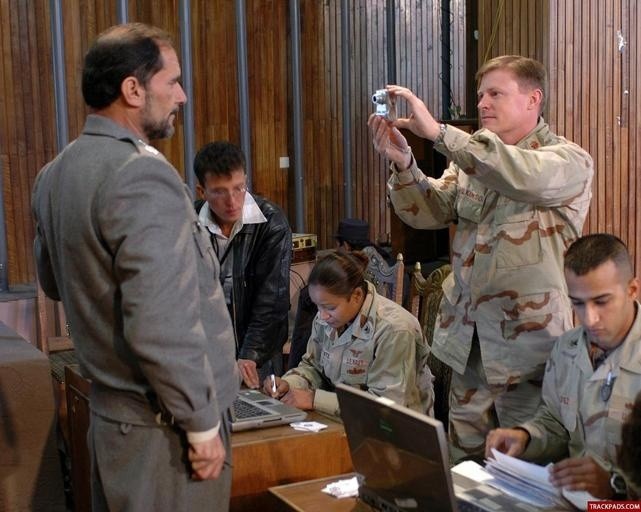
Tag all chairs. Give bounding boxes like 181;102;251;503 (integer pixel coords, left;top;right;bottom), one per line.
33;255;83;377
381;252;449;348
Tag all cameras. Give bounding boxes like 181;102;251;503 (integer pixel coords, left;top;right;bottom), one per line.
371;89;397;124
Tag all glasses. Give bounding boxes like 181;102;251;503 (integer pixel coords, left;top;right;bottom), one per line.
206;183;248;201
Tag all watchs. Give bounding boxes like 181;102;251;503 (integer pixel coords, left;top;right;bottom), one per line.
609;471;628;501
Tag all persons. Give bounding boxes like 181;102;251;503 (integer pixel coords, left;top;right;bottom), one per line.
31;24;242;512
194;142;292;389
485;234;641;501
262;251;434;423
367;55;594;466
335;218;390;252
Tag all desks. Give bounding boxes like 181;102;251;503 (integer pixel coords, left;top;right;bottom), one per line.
65;362;379;511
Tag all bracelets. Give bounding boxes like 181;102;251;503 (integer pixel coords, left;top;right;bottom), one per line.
434;124;447;145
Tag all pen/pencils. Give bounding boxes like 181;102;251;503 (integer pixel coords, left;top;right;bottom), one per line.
268;360;276;399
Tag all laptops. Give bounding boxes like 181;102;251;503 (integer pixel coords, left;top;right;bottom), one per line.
228;385;307;434
335;382;565;511
